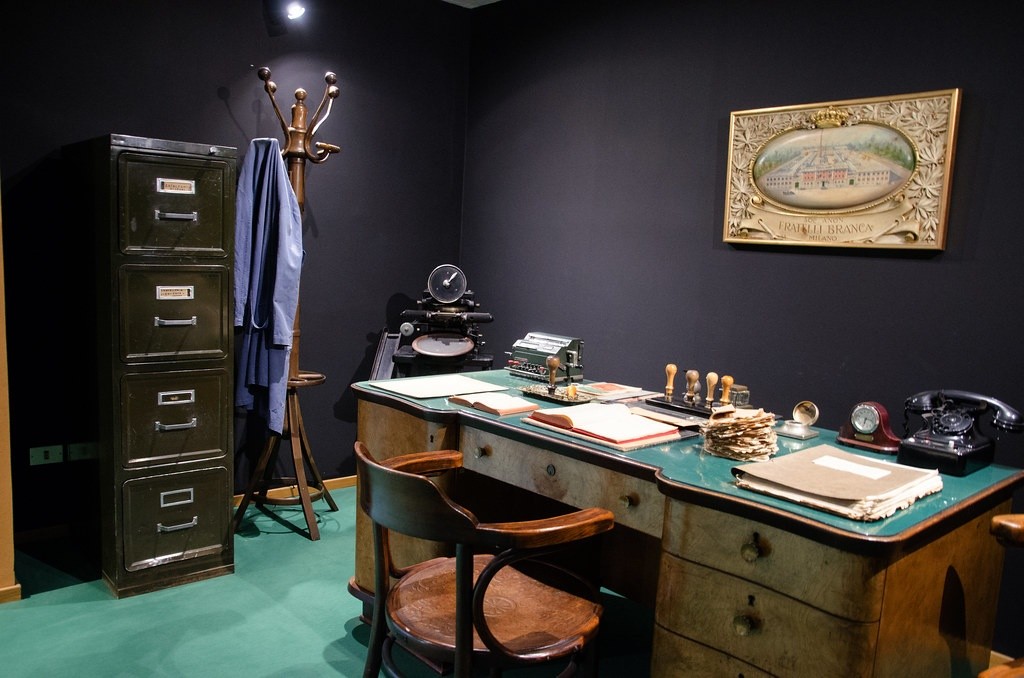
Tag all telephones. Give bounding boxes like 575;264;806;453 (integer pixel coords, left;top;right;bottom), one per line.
898;390;1024;479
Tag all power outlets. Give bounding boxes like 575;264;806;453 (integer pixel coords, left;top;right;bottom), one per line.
30;445;64;466
65;442;98;461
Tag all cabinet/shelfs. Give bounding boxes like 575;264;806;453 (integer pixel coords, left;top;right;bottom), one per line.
58;132;236;601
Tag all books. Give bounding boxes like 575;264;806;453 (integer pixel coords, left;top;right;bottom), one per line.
519;402;699;453
730;443;944;522
448;392;539;416
573;381;665;403
368;374;510;400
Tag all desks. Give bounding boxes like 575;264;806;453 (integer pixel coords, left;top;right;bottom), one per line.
347;369;1024;678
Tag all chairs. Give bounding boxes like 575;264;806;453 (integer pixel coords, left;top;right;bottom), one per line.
353;441;614;678
977;514;1024;678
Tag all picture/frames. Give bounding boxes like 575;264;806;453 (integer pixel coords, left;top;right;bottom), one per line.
722;89;962;252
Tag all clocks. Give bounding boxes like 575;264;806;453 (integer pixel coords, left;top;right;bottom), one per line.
836;401;901;456
